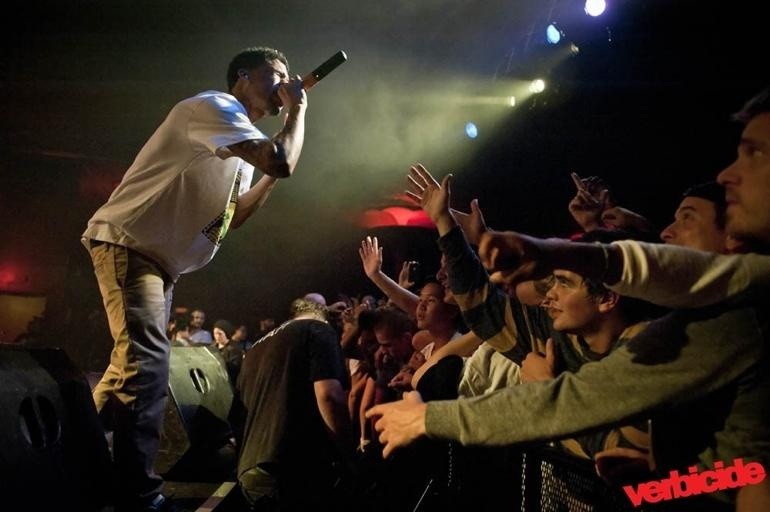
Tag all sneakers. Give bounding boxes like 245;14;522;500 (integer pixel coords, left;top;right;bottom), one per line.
114;492;176;512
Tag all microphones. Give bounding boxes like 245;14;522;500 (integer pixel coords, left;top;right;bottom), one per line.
283;50;351;102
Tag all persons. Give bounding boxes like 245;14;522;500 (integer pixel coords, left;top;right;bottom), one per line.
165;91;769;511
79;44;309;512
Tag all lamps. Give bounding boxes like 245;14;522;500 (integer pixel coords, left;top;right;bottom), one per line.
544;20;569;47
463;120;480;139
584;0;613;18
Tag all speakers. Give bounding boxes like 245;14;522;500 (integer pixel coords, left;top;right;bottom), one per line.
164;346;231;469
0;345;103;512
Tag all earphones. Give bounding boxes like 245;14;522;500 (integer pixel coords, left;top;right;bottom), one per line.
243;74;249;79
